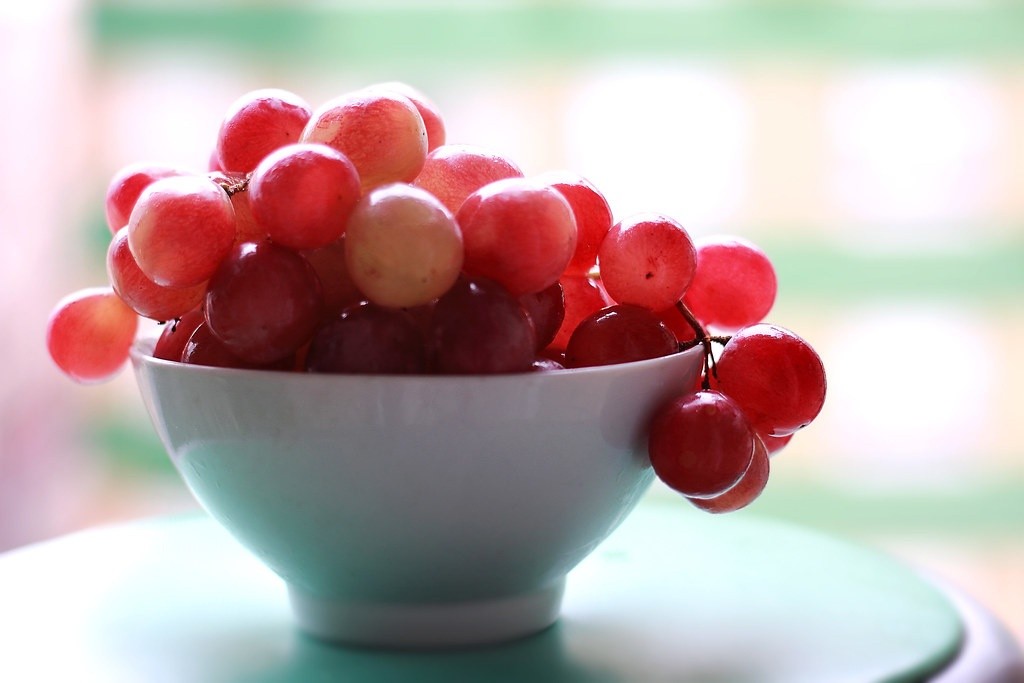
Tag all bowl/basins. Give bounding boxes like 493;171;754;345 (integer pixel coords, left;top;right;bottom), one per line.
126;320;713;656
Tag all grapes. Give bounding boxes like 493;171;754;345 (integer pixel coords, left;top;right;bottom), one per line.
49;89;826;515
